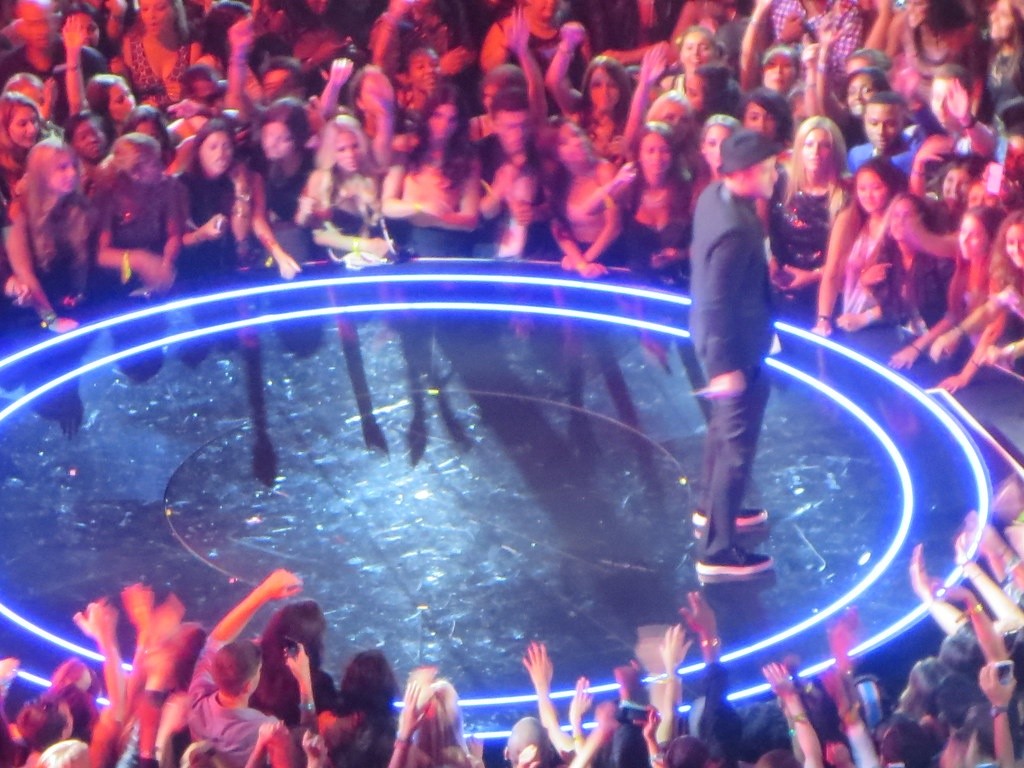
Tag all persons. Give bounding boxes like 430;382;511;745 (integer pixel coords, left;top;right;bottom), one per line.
693;133;785;576
0;481;1024;768
0;0;1024;393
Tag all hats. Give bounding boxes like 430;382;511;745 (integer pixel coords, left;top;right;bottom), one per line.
718;129;782;176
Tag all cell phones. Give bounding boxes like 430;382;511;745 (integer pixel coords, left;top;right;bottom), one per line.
285;636;298;658
622;707;649;722
988;165;1003;194
218;219;227;232
995;662;1013;686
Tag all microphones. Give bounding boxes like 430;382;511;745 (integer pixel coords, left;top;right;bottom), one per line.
690;389;711;399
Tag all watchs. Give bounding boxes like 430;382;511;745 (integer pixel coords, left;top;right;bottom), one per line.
989;704;1009;717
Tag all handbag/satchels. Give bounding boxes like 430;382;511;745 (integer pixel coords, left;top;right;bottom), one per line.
339;235;397;270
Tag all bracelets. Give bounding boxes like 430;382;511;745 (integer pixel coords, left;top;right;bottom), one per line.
236;193;254;204
579;263;587;268
0;681;12;687
961;113;975;129
64;64;81;68
956;324;966;335
841;670;853;678
393;739;411;750
969;603;982;614
816;61;829;68
646;752;663;762
414;202;423;212
660;679;669;686
193;230;201;244
816;315;832;322
352;235;359;253
270;244;280;252
972;571;982;579
299;703;316;711
775;35;787;46
971;358;980;369
700;637;721;647
790;712;807;721
41;310;57;328
1015;511;1024;522
864;309;877;326
1010;341;1020;354
122;247;132;283
910;167;927;177
911;344;922;354
572;734;583;744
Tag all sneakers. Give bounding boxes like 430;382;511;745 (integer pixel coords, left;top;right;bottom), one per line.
694;545;772;574
692;507;767;526
